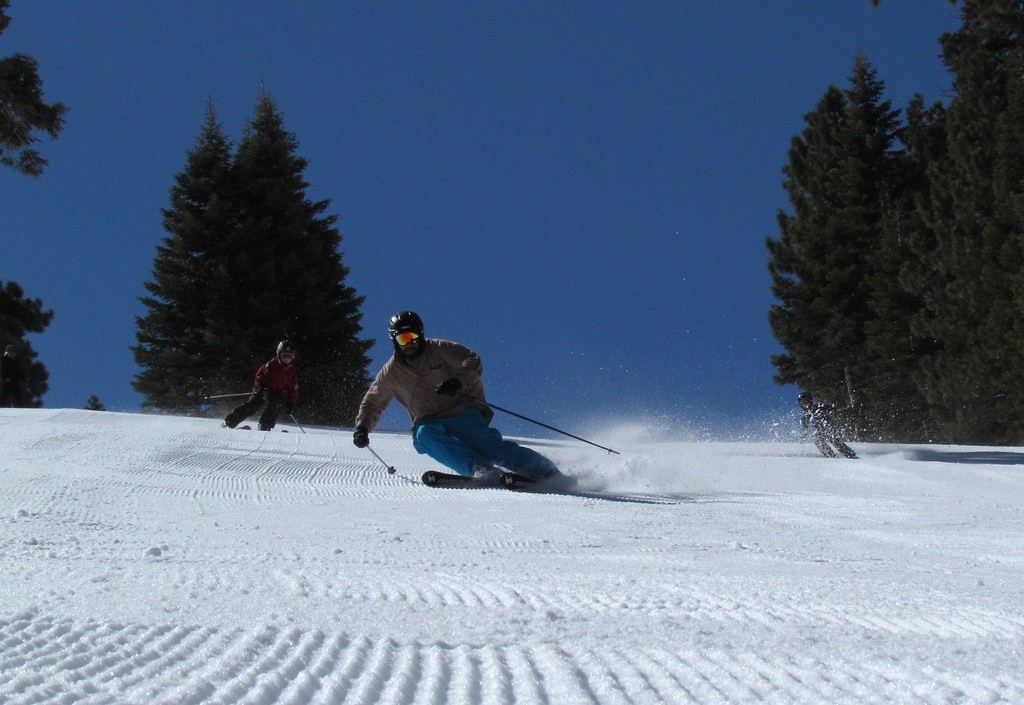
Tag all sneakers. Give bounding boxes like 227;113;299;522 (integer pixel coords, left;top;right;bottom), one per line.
470;465;505;478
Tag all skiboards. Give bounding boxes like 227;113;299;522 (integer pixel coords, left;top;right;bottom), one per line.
421;464;552;492
219;421;289;433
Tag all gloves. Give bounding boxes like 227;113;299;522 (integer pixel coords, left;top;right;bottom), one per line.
434;379;461;395
352;426;370;447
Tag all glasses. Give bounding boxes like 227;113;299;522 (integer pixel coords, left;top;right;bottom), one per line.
394;333;418;344
281;354;294;357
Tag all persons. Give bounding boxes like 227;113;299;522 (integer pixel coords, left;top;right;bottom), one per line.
797;393;859;459
221;341;300;431
353;312;578;488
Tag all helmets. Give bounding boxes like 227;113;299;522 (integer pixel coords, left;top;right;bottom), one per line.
277;341;296;358
388;310;425;345
